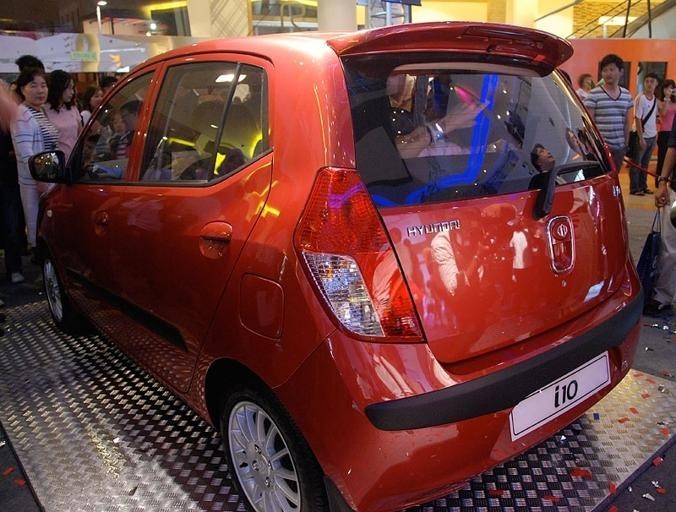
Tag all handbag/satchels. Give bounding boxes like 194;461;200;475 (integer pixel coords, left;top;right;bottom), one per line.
625;129;640;158
636;207;661;307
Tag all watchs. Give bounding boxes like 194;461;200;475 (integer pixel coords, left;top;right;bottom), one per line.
657;173;673;186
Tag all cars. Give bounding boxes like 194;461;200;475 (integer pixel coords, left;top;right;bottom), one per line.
28;20;646;512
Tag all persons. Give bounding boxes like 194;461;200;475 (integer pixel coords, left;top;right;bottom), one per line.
345;58;628;350
80;99;176;186
0;55;118;337
561;54;676;316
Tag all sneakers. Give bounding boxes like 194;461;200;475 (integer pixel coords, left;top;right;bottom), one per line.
633;188;653;196
12;272;24;283
